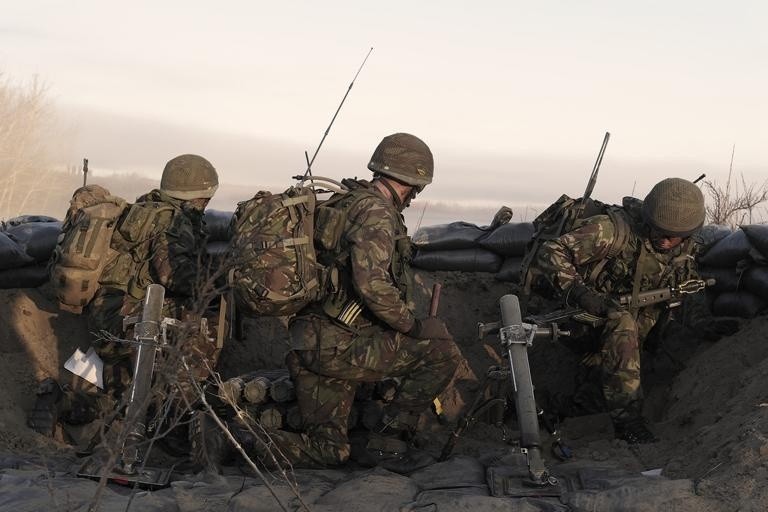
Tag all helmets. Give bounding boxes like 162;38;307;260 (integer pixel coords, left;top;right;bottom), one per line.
644;176;707;239
158;154;219;203
367;132;434;189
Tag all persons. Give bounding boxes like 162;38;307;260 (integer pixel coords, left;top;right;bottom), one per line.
188;132;462;475
27;155;223;456
535;178;707;443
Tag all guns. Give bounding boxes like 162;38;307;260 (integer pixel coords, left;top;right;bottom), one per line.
524;279;715;335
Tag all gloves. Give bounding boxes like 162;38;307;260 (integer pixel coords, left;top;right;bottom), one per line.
406;316;449;339
571;283;623;320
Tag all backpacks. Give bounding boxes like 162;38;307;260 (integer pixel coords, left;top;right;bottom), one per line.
518;194;612;296
225;185;322;320
45;182;126;314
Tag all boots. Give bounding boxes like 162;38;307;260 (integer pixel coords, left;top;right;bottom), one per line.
365;431;436;474
187;407;260;477
29;374;92;440
610;412;660;447
